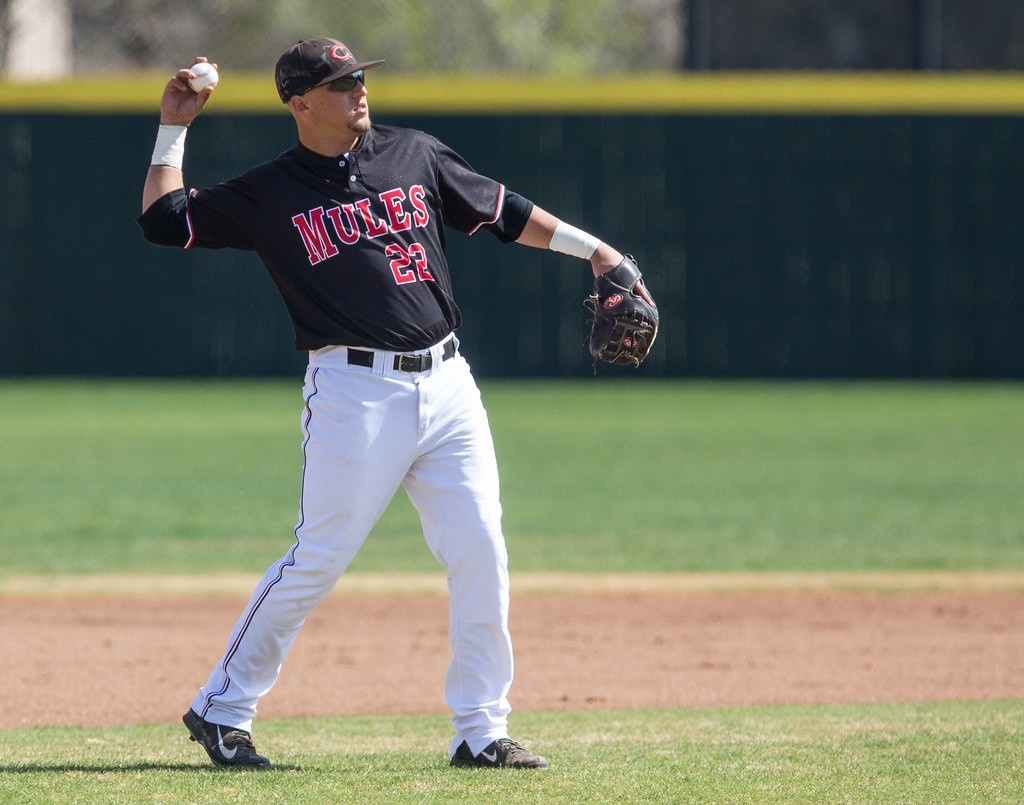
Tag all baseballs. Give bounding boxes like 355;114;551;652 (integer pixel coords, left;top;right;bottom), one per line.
188;62;220;93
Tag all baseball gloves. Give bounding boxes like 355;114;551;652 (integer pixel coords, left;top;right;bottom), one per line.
588;253;659;369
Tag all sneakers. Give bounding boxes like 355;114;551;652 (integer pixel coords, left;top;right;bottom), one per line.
182;707;270;767
450;738;548;769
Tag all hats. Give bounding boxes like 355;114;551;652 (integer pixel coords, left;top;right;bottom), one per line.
275;38;385;104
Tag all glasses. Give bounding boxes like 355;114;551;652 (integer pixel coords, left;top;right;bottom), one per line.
300;70;365;95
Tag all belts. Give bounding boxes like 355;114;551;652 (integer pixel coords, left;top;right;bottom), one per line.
347;337;455;375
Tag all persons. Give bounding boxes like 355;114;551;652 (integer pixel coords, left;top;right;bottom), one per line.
136;38;659;769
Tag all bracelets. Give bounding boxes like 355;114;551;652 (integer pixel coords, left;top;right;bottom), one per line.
549;221;603;260
150;124;187;170
